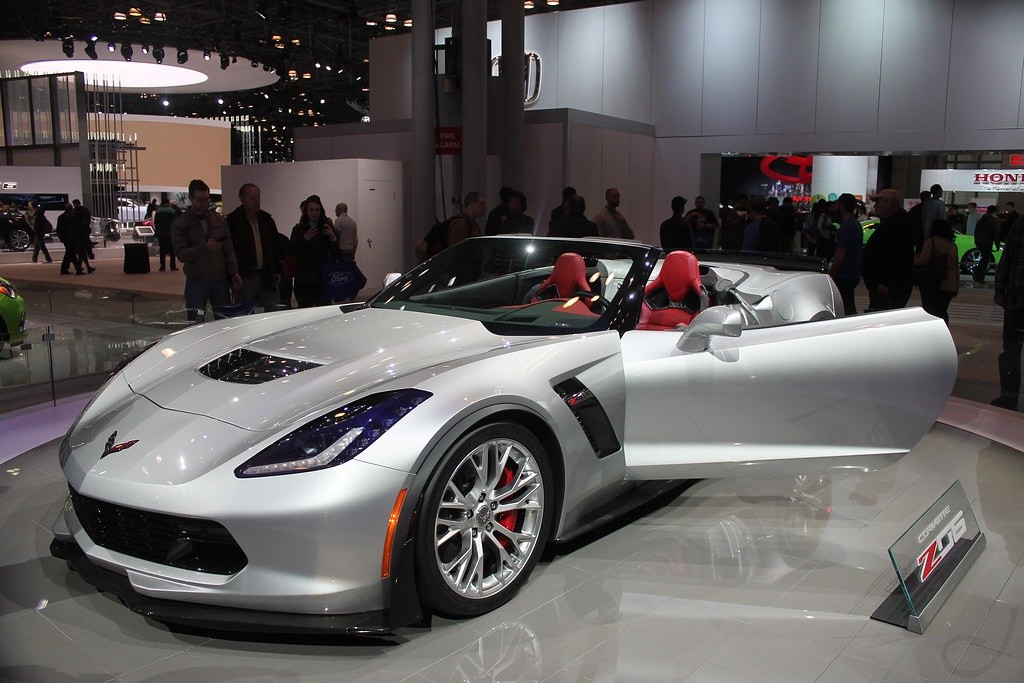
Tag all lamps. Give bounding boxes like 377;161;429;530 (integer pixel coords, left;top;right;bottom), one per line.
60;37;276;74
523;0;559;9
365;13;412;30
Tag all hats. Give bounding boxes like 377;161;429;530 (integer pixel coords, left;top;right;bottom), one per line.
299;195;325;214
868;189;905;208
671;195;688;208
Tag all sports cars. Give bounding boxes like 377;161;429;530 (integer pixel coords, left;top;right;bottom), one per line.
48;232;960;645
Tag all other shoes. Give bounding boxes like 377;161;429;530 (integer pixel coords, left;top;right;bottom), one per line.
29;260;37;263
76;268;84;271
989;395;1018;412
76;271;86;275
88;267;96;274
158;268;165;272
974;278;987;285
864;305;879;313
43;259;52;263
171;268;179;271
60;271;73;275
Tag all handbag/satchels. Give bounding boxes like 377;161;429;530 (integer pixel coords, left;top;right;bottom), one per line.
911;252;949;287
321;257;367;304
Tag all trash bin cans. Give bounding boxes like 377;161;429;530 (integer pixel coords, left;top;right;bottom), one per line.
124;242;151;273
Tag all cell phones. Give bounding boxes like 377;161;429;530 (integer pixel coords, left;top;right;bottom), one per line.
217;236;228;243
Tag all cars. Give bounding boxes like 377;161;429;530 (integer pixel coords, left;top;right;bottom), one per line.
831;216;1006;276
0;276;33;361
0;199;36;254
117;197;156;226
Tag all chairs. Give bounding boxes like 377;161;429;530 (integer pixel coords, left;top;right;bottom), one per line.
636;251;710;331
531;252;594;307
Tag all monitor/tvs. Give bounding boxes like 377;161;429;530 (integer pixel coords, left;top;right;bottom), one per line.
135;226;155;237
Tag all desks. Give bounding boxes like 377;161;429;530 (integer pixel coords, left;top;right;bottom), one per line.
123;242;153;273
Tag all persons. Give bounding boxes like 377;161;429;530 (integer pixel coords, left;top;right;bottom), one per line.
728;193;837;262
547;186;635;267
972;205;1001;283
660;196;719;250
0;200;19;212
949;202;981;236
171;179;243;325
56;199;96;275
154;199;182;271
914;218;959;324
990;214;1024;411
909;184;946;239
147;199;157;218
25;201;52;264
226;183;283;316
861;190;915;313
288;195;358;309
1000;200;1021;242
829;193;864;316
448;186;534;289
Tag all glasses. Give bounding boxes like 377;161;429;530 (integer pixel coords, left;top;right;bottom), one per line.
190;194;211;203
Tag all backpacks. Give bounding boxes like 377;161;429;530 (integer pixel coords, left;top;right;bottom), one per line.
415;214;471;266
